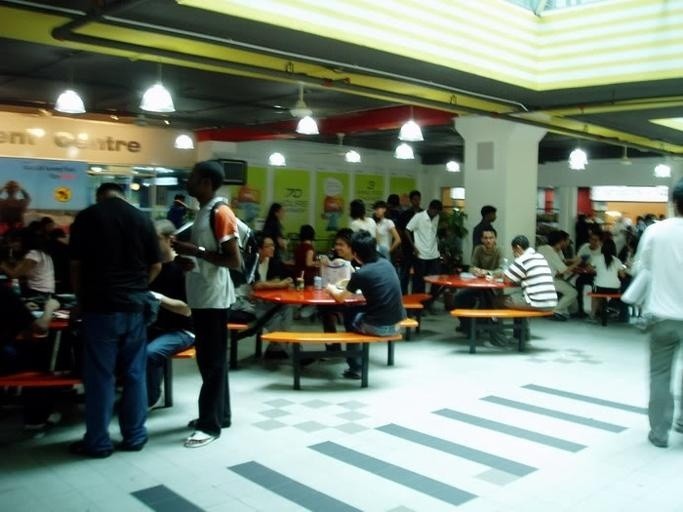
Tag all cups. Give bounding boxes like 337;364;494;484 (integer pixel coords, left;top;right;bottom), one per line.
295;278;304;288
313;276;321;290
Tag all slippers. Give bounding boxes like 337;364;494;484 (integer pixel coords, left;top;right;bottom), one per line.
184;431;217;448
188;418;199;428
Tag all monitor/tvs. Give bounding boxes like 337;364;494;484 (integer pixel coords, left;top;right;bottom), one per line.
218;157;249;187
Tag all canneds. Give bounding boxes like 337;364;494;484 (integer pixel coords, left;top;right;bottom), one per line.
295;278;305;292
314;276;322;289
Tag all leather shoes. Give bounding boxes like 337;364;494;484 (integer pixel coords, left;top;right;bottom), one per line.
551;313;567;322
648;430;668;448
676;424;683;432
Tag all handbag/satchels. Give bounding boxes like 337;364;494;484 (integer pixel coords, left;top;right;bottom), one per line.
232;283;256;314
621;269;653;307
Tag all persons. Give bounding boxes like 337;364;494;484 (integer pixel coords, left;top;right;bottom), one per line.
0;181;89;434
473;205;497;323
230;190;444;379
630;180;683;447
490;235;558;347
66;183;149;457
454;228;505;332
112;218;198;413
168;194;192;242
168;161;241;448
535;206;665;321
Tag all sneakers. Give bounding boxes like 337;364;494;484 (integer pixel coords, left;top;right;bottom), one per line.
300;357;319;369
343;367;362;378
148;389;163;410
265;348;290;362
63;440;115;459
122;436;149;452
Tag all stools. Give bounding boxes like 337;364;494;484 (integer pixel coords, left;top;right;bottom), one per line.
227;322;249;368
388;317;418;366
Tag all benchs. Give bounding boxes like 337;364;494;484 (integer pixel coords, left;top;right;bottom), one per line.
261;331;403;389
400;294;433;334
0;369;84;388
587;292;628;325
164;345;197;407
451;309;554;352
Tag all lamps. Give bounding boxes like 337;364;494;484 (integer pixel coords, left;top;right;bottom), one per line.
53;90;86;114
653;156;674;179
173;134;195;150
270;82;461;173
139;64;176;113
567;139;591;171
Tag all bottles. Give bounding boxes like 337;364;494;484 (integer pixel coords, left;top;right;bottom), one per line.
11;277;20;294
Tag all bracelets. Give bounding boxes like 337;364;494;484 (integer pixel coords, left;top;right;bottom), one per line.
196;246;206;259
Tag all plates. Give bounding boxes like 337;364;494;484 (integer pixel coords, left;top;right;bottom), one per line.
31;311;69;320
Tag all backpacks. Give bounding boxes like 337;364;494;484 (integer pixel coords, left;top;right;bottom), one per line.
230;217;260;287
